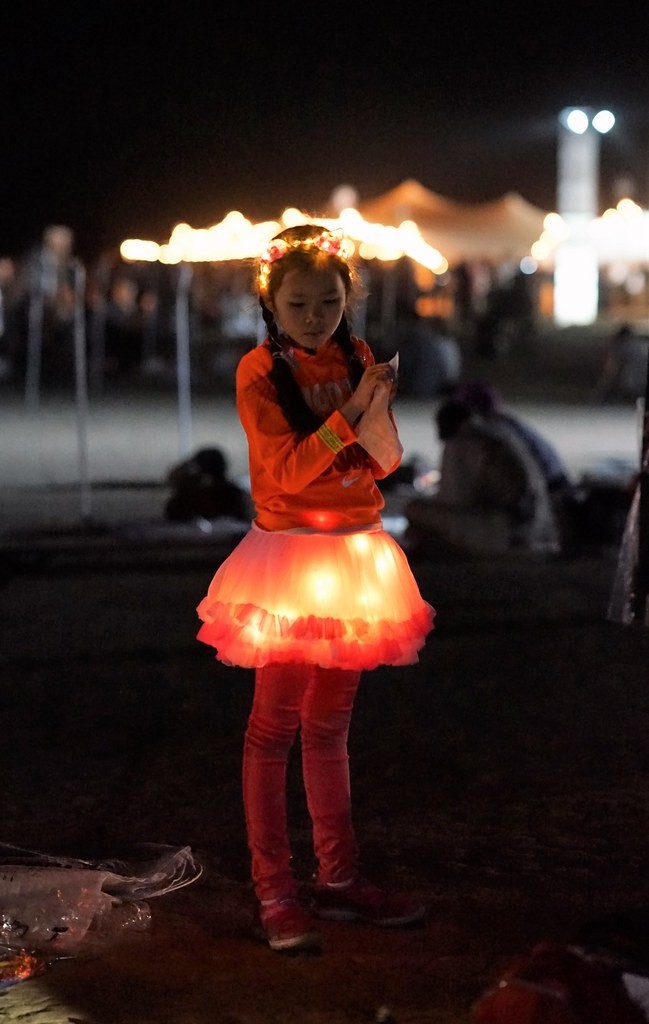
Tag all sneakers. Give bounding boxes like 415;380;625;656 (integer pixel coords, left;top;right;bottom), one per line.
311;874;425;927
258;894;322;950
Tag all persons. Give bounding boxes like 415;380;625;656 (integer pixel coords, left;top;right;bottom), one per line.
196;225;436;951
400;378;576;563
165;447;251;523
419;317;461;393
